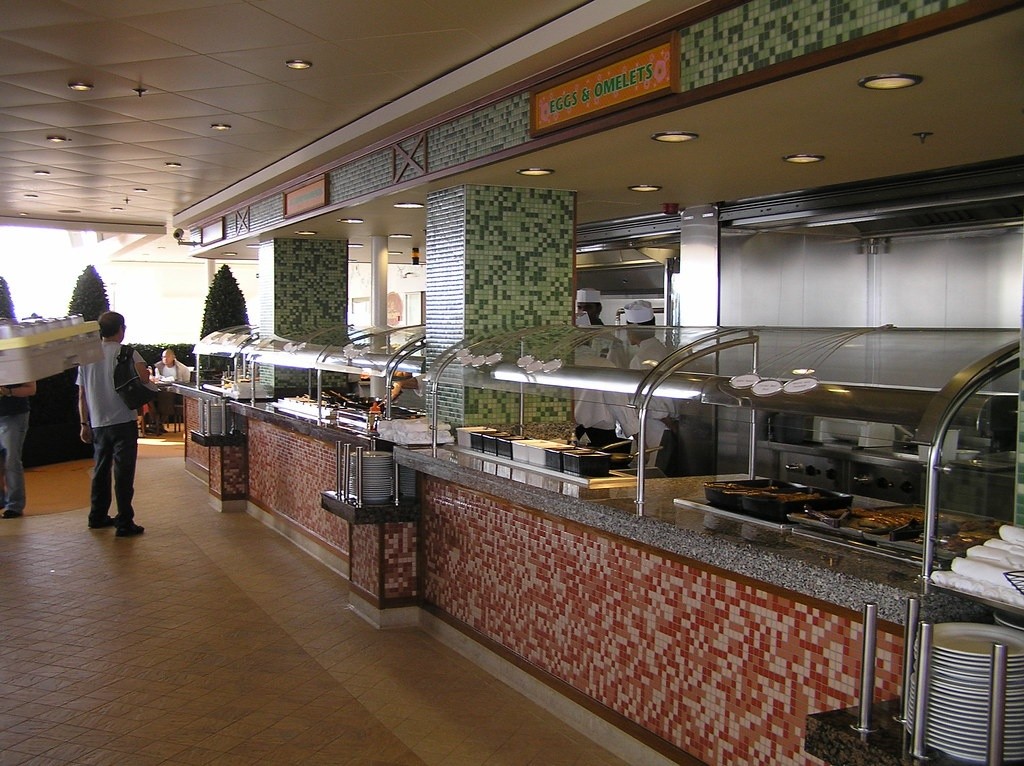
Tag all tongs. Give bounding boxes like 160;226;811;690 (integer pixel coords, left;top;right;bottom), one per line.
801;504;853;526
890;518;962;541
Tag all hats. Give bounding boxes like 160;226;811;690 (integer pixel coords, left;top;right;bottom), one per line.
575;288;601;303
625;300;654;323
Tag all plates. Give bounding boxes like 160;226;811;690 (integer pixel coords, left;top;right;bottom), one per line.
905;622;1024;762
203;404;231;435
349;451;416;505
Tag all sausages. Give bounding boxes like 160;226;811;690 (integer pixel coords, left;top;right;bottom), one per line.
849;506;944;529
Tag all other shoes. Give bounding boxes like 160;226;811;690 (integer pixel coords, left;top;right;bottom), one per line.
115;521;144;537
89;516;115;529
2;510;21;518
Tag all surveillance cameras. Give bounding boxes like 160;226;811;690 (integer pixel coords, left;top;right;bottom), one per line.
174;228;184;239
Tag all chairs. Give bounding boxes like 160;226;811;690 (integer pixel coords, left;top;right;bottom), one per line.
137;370;196;439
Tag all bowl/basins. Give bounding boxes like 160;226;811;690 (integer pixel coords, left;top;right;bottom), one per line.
956;449;980;460
917;445;929;462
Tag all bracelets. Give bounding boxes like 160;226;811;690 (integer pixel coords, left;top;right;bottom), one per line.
80;422;89;426
7;388;12;396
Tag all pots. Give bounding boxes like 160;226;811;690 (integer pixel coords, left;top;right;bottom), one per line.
609;446;664;467
578;440;633;452
338;395;383;406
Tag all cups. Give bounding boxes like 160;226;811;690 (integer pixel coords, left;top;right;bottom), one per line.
941;430;958;466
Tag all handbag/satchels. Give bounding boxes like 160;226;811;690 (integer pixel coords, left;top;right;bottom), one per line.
113;345;156;410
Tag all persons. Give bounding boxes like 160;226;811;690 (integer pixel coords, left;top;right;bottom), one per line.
624;300;679;469
0;317;36;518
385;339;426;403
574;288;650;467
75;312;151;536
138;350;190;433
